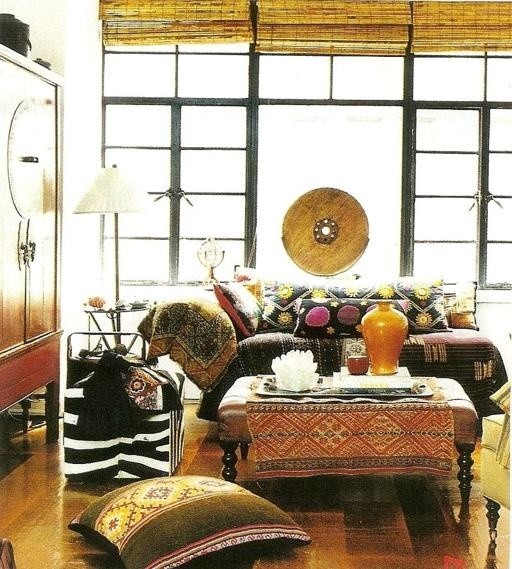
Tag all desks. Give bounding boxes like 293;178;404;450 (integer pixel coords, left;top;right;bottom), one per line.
80;298;155;354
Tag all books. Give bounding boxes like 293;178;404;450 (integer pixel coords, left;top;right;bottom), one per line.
332;366;414;394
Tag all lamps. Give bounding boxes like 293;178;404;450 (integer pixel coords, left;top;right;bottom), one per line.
75;167;156;356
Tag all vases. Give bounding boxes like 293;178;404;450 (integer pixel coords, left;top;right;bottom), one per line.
361;300;410;376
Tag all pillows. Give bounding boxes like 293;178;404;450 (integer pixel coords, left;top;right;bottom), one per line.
212;279;262;335
258;281;447;334
442;281;479;331
295;297;411;339
66;476;313;569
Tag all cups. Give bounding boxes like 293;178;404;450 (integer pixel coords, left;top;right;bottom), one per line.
347;356;370;375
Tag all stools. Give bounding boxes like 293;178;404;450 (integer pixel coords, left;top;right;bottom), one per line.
64;370;186;479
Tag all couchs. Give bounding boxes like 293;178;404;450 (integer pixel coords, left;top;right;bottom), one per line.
483;407;510;554
137;282;507;437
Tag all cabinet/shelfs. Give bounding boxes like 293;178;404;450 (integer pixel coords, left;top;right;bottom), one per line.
0;42;63;444
